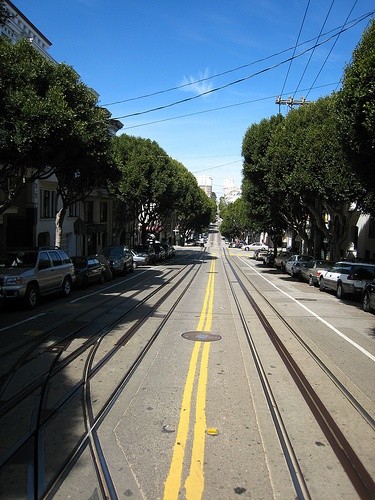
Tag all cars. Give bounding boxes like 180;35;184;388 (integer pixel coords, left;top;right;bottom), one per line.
241;242;269;252
252;248;298;271
183;231;209;247
360;278;375;314
71;256;107;290
128;242;176;269
222;237;245;248
298;260;333;286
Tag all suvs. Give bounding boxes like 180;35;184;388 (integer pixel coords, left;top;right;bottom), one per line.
0;244;76;311
284;254;315;278
317;260;375;299
97;244;134;277
